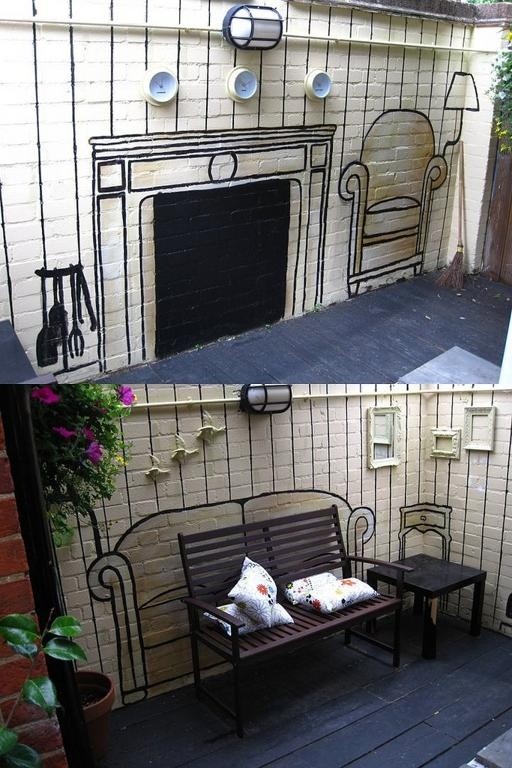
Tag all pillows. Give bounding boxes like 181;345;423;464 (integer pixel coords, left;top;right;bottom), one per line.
207;556;379;637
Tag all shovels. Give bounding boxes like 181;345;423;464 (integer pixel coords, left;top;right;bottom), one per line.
36;267;67;367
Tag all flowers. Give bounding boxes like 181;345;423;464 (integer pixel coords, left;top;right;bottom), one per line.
40;385;139;539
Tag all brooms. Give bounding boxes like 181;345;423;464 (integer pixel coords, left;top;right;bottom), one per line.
435;140;465;292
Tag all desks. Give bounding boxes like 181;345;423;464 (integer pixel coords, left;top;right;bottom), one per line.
368;552;487;661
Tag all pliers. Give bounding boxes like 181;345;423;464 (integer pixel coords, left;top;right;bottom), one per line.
75;264;96;331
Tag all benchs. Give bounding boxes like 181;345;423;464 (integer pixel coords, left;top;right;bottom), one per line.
178;505;414;738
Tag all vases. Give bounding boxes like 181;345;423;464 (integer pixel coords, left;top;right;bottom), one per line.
70;669;114;768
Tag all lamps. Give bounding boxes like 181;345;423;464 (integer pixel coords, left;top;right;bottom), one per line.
222;5;282;52
241;383;290;417
